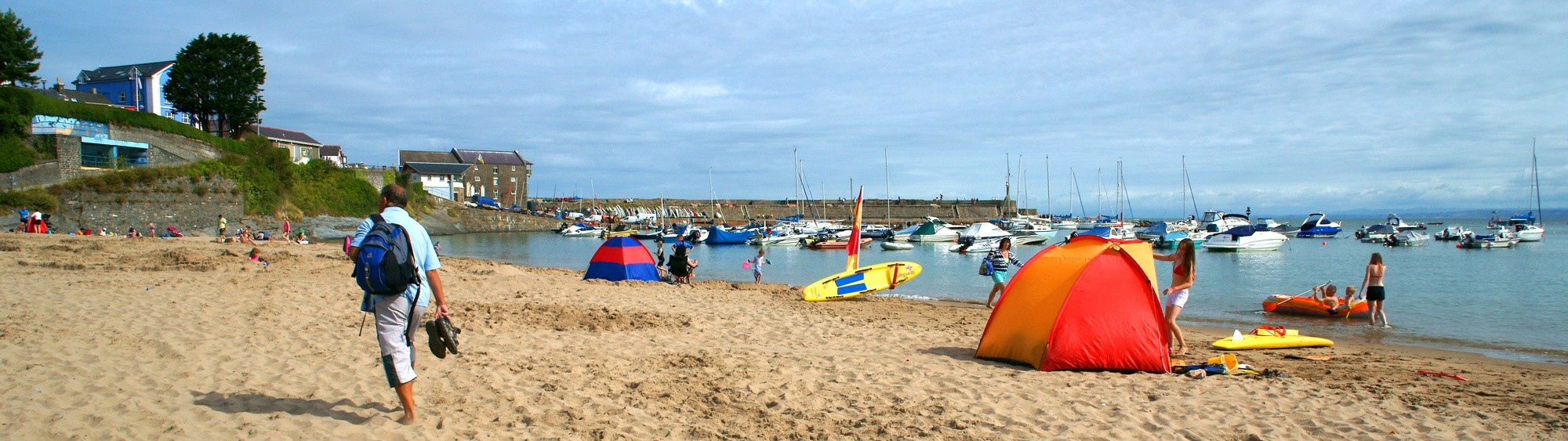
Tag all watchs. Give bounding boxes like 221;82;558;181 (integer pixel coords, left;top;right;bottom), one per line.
1315;291;1318;293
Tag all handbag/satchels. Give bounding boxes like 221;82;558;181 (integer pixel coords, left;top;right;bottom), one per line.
979;259;993;275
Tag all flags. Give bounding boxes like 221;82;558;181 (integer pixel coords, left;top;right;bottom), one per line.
477;154;484;164
845;189;862;272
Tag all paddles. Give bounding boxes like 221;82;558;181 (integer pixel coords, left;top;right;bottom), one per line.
1262;279;1332;312
1344;231;1355;239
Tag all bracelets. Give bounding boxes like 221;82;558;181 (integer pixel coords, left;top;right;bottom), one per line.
1172;287;1176;291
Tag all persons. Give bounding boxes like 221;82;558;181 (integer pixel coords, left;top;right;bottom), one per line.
838;196;846;204
991;196;997;201
746;248;768;284
766;226;772;235
936;194;943;201
1077;216;1079;222
1153;238;1196;356
247;248;261;261
1188;214;1195;224
654;234;698;285
956;197;978;205
986;237;1024;309
18;205;307;245
646;216;657;227
757;212;772;219
1313;252;1387;327
348;183;449;425
625;198;633;203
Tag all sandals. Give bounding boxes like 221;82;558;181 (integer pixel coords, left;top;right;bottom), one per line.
437;316;462;354
426;320;446;359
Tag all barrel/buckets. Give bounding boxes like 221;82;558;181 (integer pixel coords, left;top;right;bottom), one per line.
742;259;750;270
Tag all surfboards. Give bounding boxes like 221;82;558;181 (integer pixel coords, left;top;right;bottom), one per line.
802;261;923;302
1211;330;1335;351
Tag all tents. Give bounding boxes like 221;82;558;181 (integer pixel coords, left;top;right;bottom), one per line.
25;215;47;233
974;235;1172;374
582;234;662;281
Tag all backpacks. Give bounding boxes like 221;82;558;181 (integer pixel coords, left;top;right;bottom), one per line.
355;212;422;296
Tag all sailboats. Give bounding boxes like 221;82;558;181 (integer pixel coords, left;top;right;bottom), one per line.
464;146;1552;255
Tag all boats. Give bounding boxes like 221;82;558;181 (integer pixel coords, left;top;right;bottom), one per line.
1261;294;1380;320
